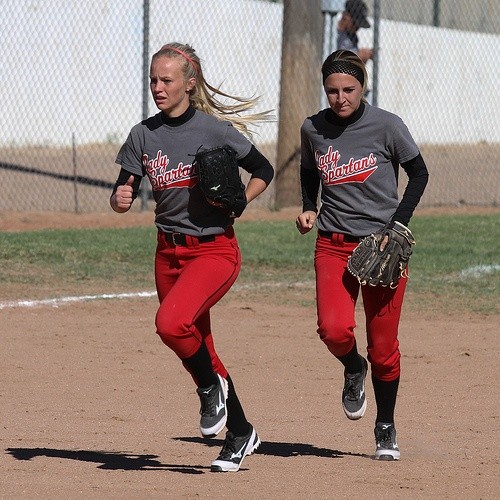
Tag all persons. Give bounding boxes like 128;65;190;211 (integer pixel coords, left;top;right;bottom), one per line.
293;50;429;462
323;0;375;111
109;42;275;472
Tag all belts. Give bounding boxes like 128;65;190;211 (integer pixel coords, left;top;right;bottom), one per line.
319;230;360;243
165;234;215;244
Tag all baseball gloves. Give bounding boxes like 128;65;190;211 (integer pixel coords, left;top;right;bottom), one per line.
195;145;248;219
346;221;417;289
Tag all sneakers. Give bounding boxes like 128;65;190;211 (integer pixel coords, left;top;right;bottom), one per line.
342;354;368;420
374;422;400;460
196;374;229;438
211;422;261;471
